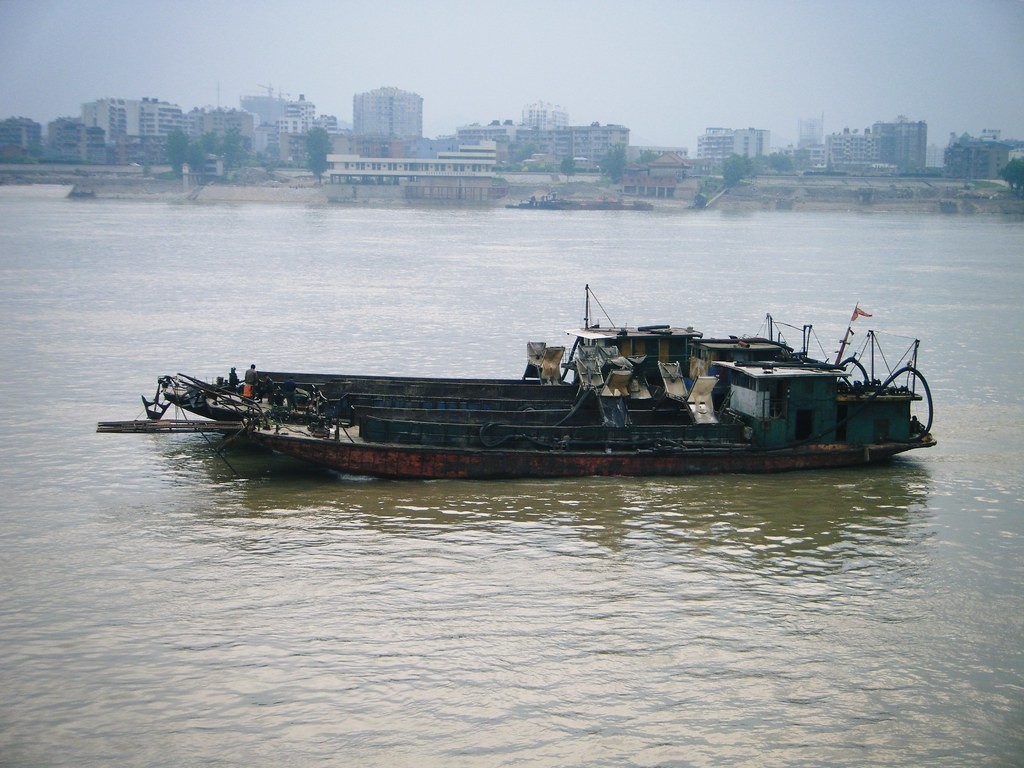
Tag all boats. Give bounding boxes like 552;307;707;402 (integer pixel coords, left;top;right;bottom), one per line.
93;279;943;481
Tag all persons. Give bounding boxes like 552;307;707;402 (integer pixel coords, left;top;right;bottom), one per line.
280;376;298;410
254;374;273;403
245;364;256;386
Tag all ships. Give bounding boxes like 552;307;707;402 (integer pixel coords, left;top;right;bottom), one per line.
318;137;512;201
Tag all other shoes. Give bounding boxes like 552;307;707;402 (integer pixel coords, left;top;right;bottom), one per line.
257;401;262;403
254;398;259;400
